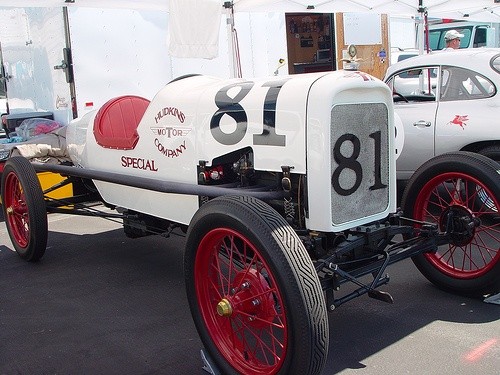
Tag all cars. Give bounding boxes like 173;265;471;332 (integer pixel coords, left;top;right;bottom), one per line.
380;49;500;222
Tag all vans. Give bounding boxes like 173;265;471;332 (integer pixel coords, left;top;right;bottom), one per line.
421;21;490;55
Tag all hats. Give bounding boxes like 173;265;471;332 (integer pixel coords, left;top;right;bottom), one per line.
445;30;464;41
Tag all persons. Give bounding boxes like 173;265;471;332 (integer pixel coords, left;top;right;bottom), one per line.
432;30;464;87
